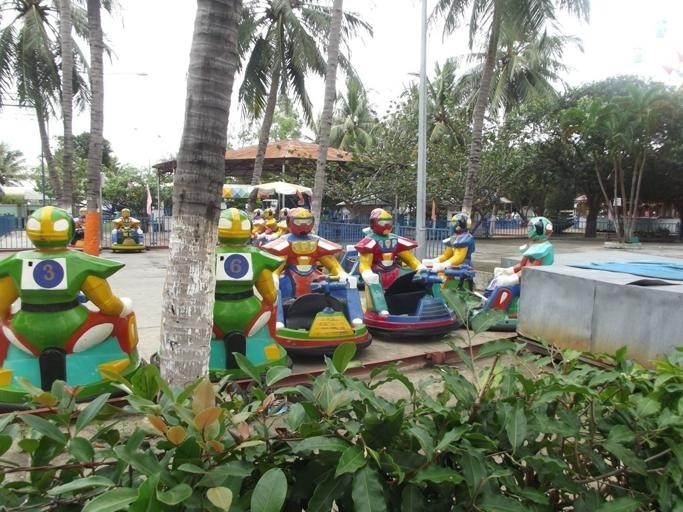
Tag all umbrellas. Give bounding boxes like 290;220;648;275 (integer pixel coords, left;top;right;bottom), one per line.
258;180;313;209
